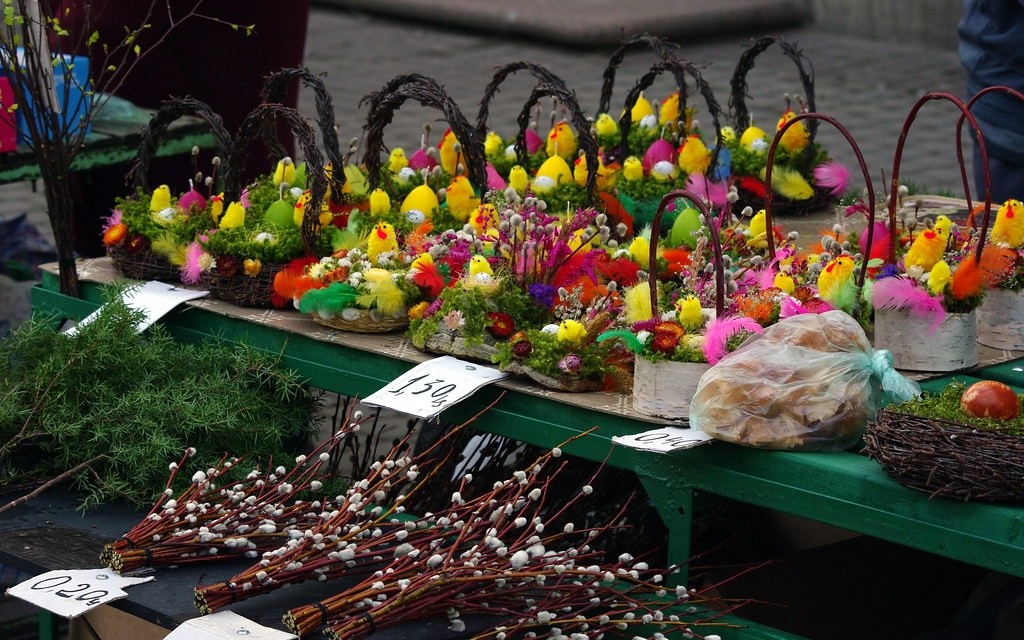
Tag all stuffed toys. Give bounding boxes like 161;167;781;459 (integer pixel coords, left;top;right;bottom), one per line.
150;184;170;211
773;254;854;303
367;127;504;277
507;93;805;189
749;210;767;238
991;200;1024;248
274;159;296;186
212;192;246;229
679;296;703;321
906;215;954;274
558;319;588;342
294;163;351;231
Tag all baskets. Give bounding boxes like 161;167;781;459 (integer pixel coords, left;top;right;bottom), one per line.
108;37;836;309
310;304;411;333
868;409;1023;501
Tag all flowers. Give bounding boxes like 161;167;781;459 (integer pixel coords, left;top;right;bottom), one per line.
97;88;1024;384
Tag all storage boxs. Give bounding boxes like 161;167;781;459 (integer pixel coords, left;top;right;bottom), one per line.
1;46;94;145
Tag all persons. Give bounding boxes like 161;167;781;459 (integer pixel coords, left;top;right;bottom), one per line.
957;0;1024;205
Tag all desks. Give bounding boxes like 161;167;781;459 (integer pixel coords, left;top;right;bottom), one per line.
0;102;227;198
30;257;1024;640
1;495;605;640
